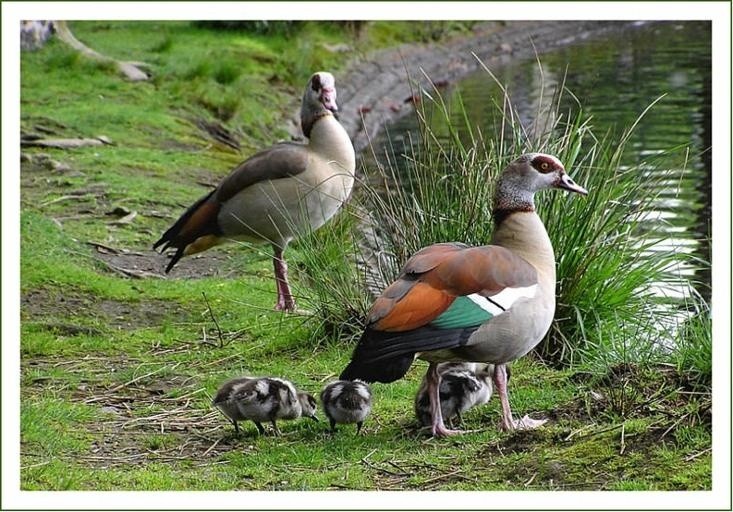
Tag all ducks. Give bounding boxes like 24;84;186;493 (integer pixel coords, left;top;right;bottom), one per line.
152;70;356;315
339;152;588;437
211;378;258;436
319;381;373;437
415;361;510;430
235;377;319;438
